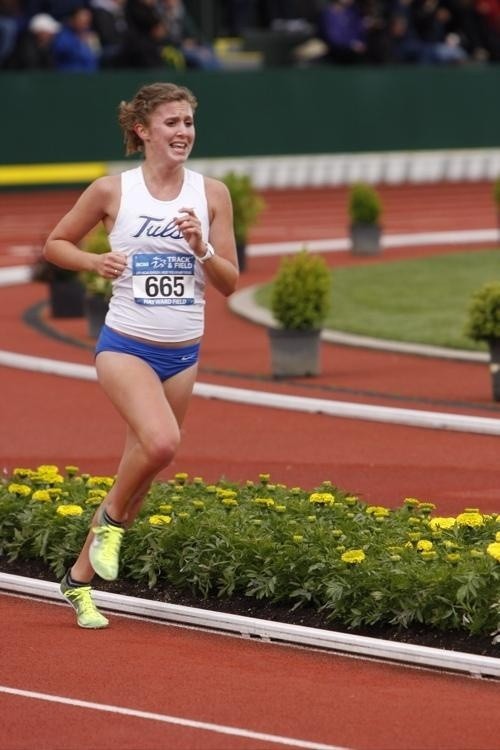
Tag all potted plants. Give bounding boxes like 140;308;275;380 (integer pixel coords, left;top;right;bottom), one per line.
26;224;113;339
217;171;270;276
463;279;500;402
343;177;384;257
265;242;333;380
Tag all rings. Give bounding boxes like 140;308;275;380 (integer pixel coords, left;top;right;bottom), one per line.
114;269;118;275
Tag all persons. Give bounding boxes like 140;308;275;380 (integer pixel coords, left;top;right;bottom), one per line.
1;1;499;72
43;82;239;629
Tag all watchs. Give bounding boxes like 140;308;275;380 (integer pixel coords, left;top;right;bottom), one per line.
193;242;215;265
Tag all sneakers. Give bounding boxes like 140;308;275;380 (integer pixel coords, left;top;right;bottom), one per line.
88;503;126;582
58;568;109;629
